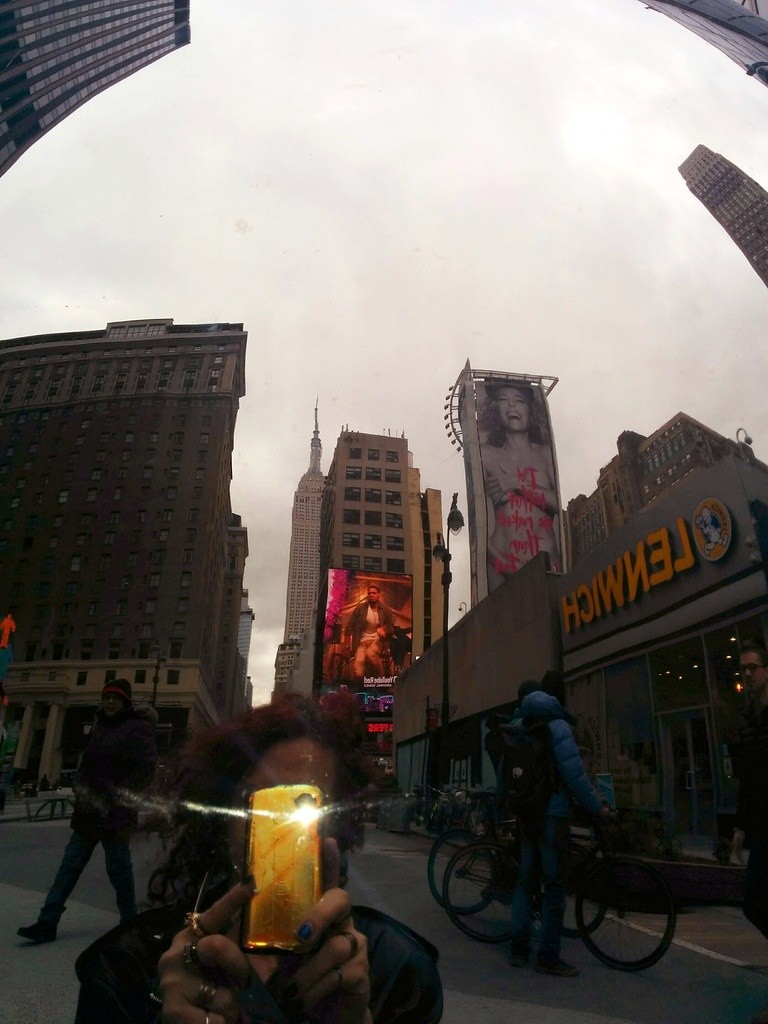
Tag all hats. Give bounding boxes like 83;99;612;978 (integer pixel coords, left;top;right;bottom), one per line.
101;677;132;702
516;679;542;703
541;670;566;709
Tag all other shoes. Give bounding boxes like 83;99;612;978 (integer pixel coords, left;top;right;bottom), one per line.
533;956;580;978
16;919;57;942
506;947;530;967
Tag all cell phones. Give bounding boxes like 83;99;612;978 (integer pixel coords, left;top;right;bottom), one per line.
239;784;323;953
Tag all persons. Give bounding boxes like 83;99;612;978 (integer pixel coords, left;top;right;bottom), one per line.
492;671;610;976
40;773;49;791
17;678;157;941
475;380;565;593
344;585;392;680
74;697;444;1024
726;636;768;1024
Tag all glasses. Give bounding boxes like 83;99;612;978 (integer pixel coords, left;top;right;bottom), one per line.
737;662;766;671
103;693;123;702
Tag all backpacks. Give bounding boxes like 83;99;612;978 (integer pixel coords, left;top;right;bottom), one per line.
500;720;562;819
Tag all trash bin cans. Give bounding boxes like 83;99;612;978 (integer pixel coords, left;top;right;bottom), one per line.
375;794;416;834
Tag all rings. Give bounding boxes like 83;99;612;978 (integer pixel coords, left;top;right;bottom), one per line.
341;932;358;957
185;913;210;935
205;1016;209;1023
184;939;199;964
336;968;342;982
198;982;217;1008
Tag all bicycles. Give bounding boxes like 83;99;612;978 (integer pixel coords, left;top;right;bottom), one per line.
411;783;502;843
442;798;677;971
427;791;610;915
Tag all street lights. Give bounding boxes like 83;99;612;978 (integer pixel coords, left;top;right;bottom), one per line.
433;492;466;727
148;645;167;708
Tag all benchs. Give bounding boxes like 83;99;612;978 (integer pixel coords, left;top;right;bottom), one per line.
22;794;69;820
